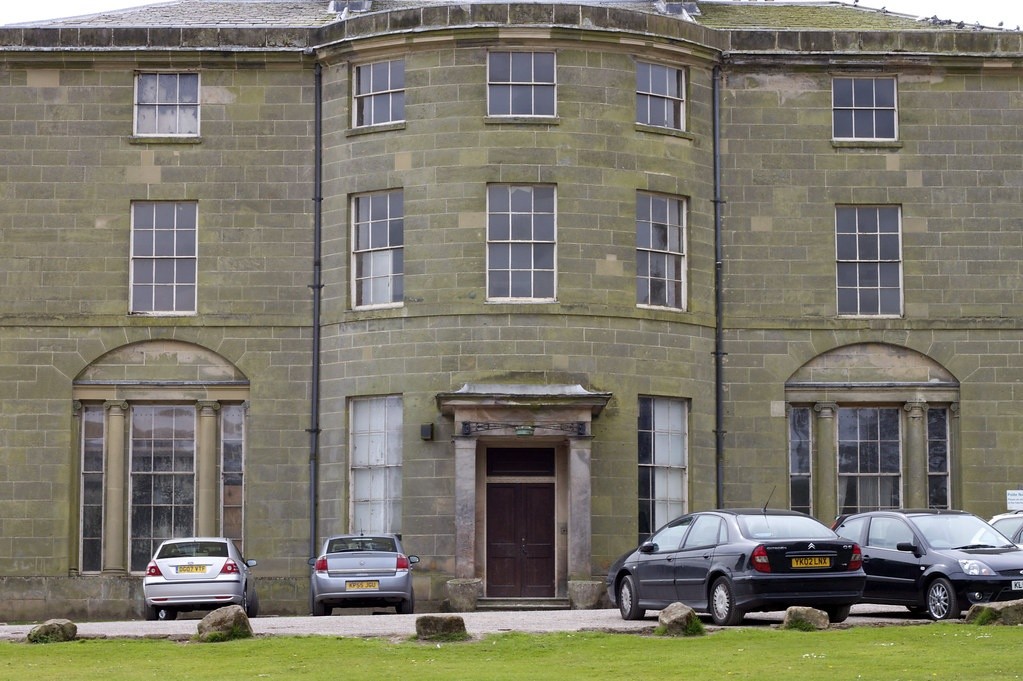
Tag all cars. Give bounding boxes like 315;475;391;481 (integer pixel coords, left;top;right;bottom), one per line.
971;511;1023;550
307;532;421;617
142;537;259;621
606;508;867;630
829;507;1023;620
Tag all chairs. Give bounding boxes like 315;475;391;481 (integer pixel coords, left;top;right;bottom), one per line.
373;544;390;551
335;546;348;550
